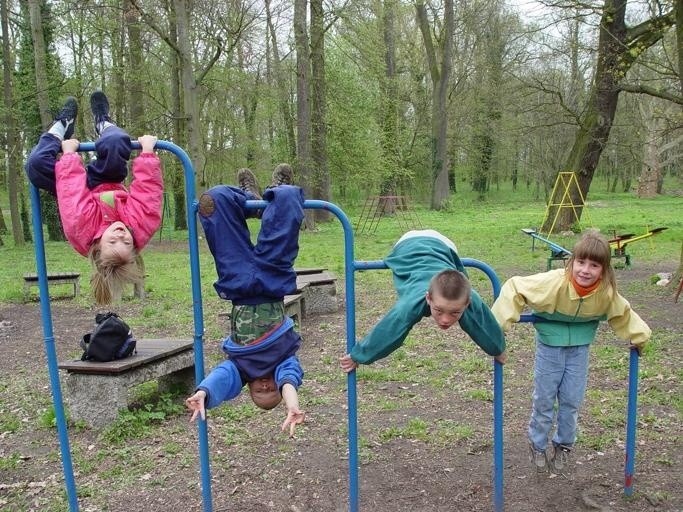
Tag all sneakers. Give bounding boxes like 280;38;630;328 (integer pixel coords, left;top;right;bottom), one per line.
88;90;109;133
552;438;574;476
56;97;78;143
237;167;263;217
268;163;294;187
527;439;549;477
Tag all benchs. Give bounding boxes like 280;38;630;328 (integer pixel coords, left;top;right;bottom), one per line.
218;267;339;337
21;271;81;303
54;337;199;428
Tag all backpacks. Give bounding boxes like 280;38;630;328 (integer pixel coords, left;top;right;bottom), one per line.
80;312;136;364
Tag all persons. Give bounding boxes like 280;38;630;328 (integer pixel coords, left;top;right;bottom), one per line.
340;228;507;373
24;89;162;306
185;165;308;441
491;232;651;473
